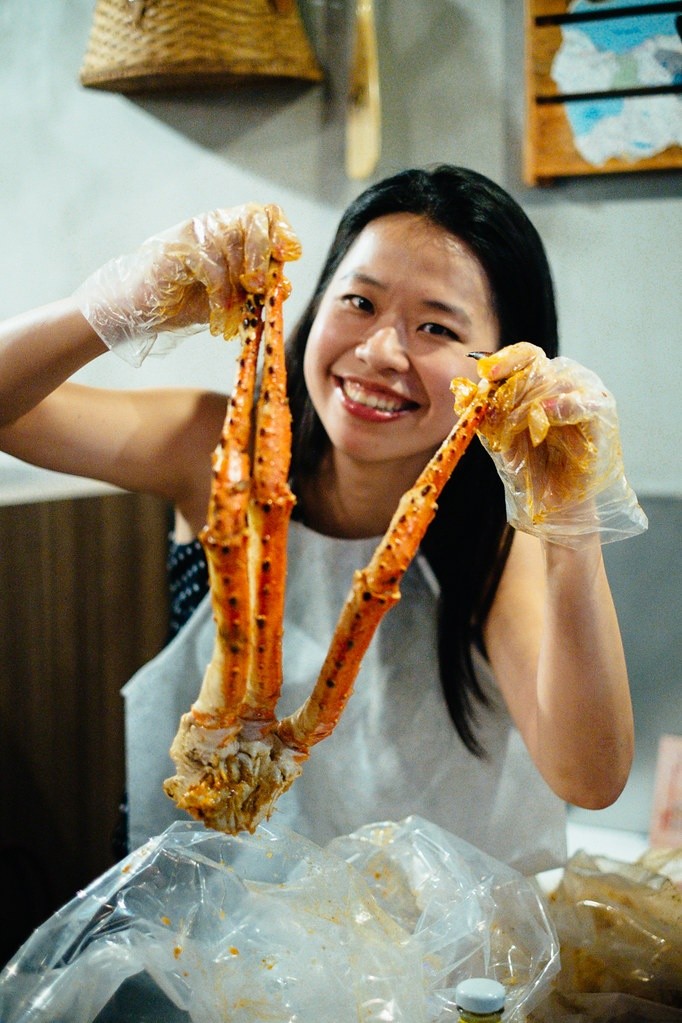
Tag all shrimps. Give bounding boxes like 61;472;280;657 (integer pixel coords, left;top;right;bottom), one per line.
159;260;500;833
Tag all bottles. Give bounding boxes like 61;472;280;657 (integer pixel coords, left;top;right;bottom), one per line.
455;977;505;1023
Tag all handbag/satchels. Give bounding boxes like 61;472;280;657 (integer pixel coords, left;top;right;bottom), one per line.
79;0;322;89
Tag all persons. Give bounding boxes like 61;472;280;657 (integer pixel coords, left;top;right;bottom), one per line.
0;163;635;899
